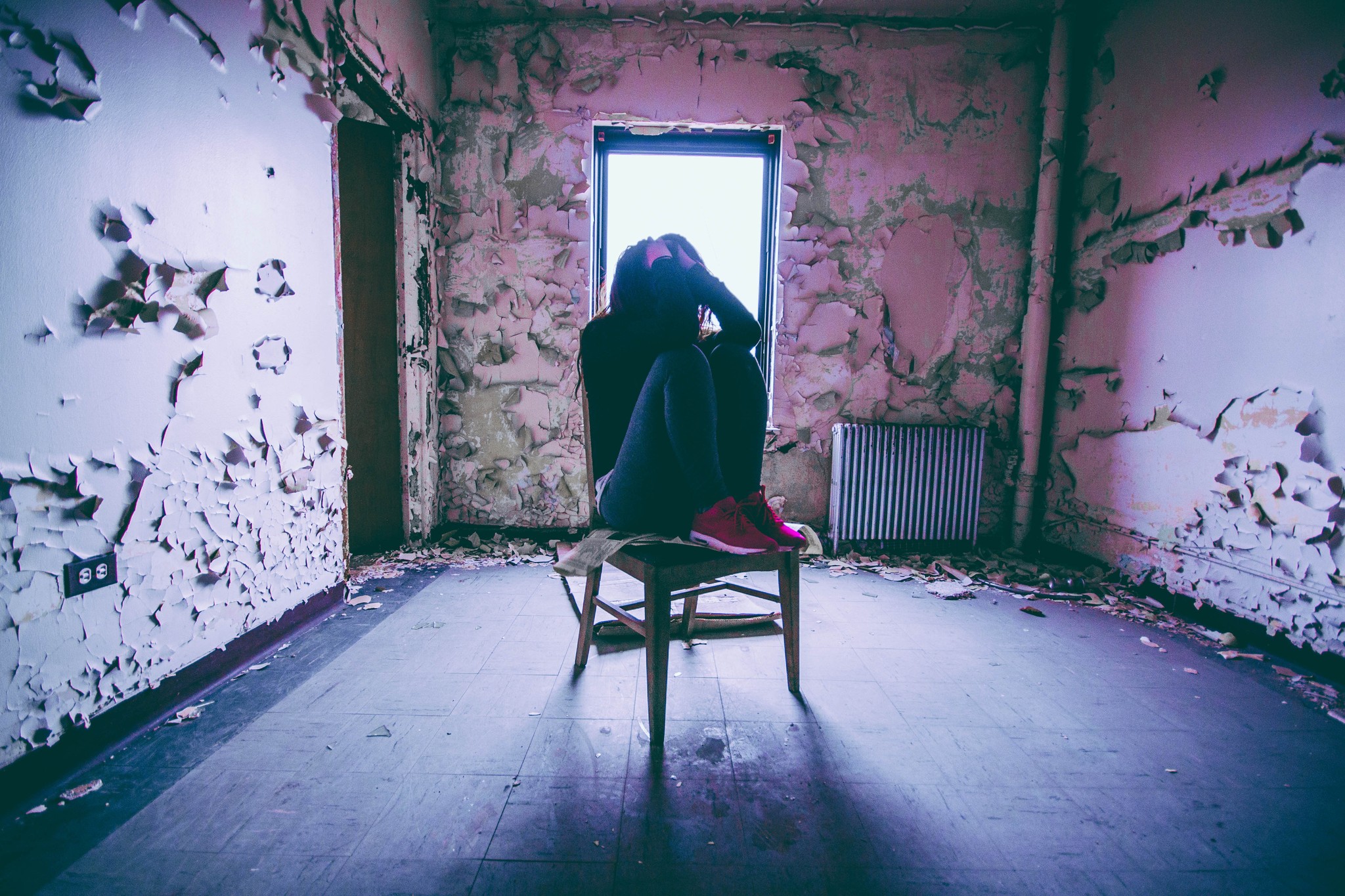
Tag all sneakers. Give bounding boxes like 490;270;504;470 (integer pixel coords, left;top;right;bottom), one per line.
689;496;778;555
739;484;806;549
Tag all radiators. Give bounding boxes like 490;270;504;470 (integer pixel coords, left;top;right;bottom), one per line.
824;422;987;557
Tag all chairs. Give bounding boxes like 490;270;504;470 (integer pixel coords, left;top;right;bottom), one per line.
574;361;801;745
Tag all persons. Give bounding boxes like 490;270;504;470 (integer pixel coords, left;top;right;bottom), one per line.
575;233;808;554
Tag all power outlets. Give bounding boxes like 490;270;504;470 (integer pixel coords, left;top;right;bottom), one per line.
64;551;118;598
